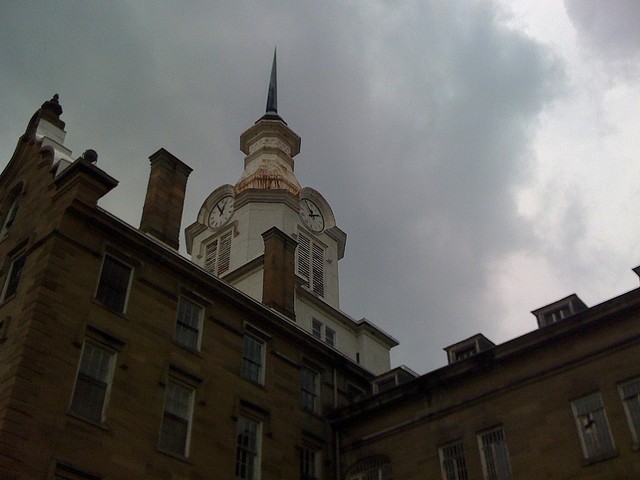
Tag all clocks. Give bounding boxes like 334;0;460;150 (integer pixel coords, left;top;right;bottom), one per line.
206;194;236;230
298;197;325;233
